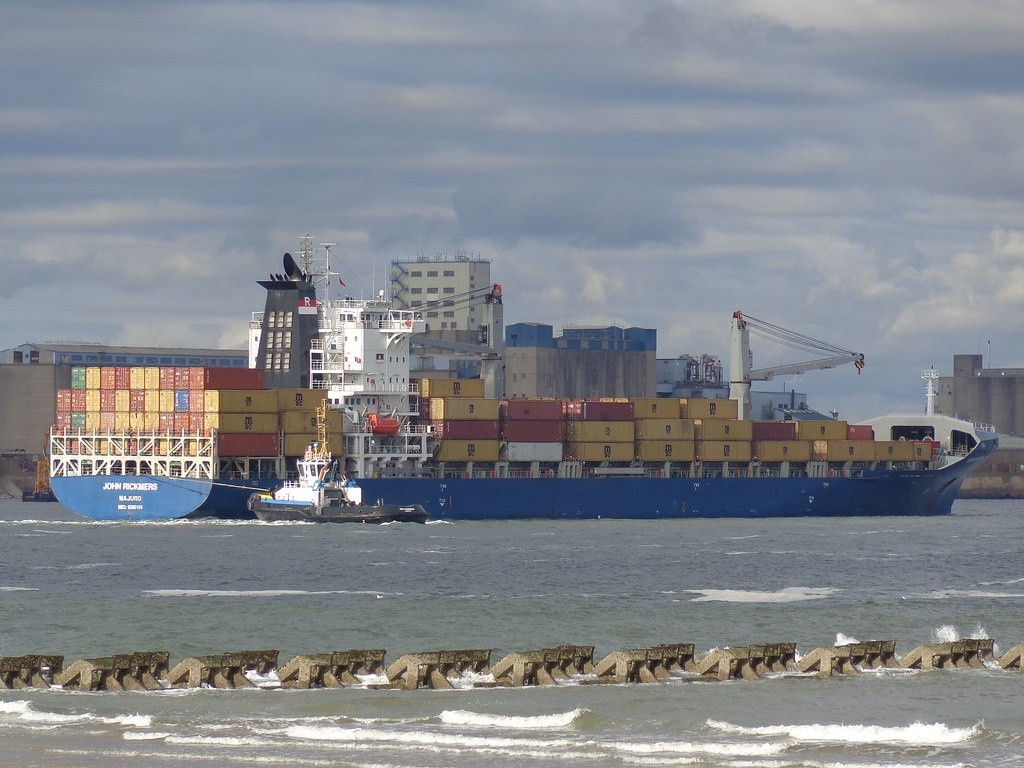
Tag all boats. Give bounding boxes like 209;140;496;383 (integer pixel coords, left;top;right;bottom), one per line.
248;440;430;524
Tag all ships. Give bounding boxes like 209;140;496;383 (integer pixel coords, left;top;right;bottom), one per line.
47;289;998;523
